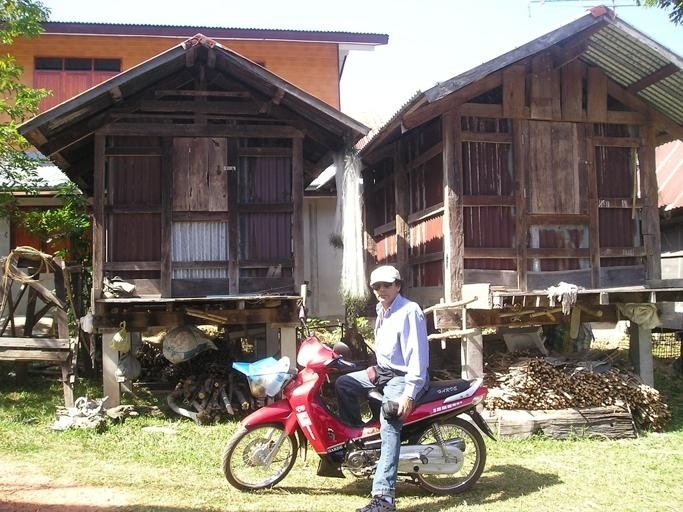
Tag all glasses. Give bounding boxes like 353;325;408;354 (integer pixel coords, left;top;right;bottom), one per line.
371;281;393;291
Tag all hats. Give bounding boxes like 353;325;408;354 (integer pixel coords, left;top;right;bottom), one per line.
368;264;401;287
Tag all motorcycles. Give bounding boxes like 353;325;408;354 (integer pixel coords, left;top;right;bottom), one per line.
221;283;496;496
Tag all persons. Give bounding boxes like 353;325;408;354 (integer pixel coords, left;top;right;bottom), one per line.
333;264;431;512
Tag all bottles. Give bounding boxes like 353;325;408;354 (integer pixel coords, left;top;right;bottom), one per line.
264;356;289;398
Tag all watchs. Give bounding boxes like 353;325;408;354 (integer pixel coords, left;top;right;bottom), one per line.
403;395;415;402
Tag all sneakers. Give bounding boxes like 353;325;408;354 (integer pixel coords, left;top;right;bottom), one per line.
354;494;396;512
